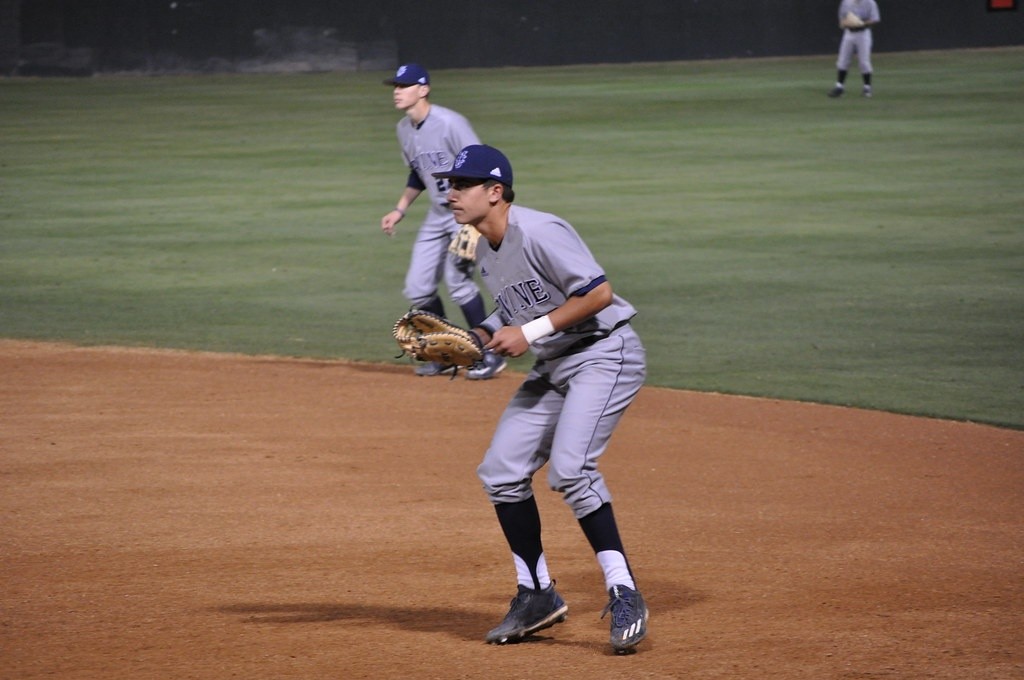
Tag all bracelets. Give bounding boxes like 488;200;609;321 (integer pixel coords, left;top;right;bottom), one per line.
521;315;557;344
393;207;406;218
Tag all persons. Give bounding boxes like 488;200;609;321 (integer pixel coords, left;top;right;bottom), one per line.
381;61;509;380
827;0;880;102
391;143;650;654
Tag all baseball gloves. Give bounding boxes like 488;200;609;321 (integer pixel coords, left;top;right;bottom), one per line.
392;309;486;368
446;224;481;262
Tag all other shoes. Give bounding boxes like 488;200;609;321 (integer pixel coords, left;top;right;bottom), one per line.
862;86;873;97
828;86;844;97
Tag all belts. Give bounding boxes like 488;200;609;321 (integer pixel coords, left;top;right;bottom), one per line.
551;318;630;359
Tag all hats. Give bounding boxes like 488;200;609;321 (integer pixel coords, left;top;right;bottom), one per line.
431;144;514;187
382;63;431;85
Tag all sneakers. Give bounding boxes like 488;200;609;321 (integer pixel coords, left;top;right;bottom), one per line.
466;349;508;379
415;359;462;380
601;584;649;653
487;580;567;644
